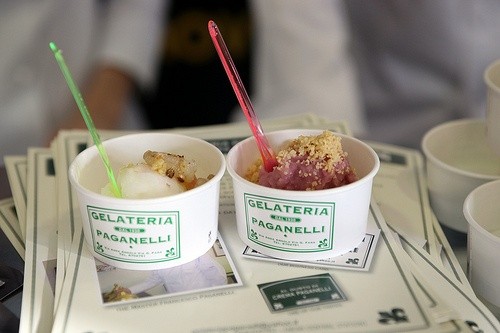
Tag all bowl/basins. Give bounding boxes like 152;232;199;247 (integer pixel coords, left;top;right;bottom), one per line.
69;133;225;271
462;180;500;308
225;128;380;261
483;60;500;118
422;118;500;232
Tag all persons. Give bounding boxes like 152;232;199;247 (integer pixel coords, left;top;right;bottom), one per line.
0;0;500;167
116;252;228;295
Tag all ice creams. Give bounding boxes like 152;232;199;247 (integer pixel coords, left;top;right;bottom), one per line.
243;130;357;191
97;150;213;198
